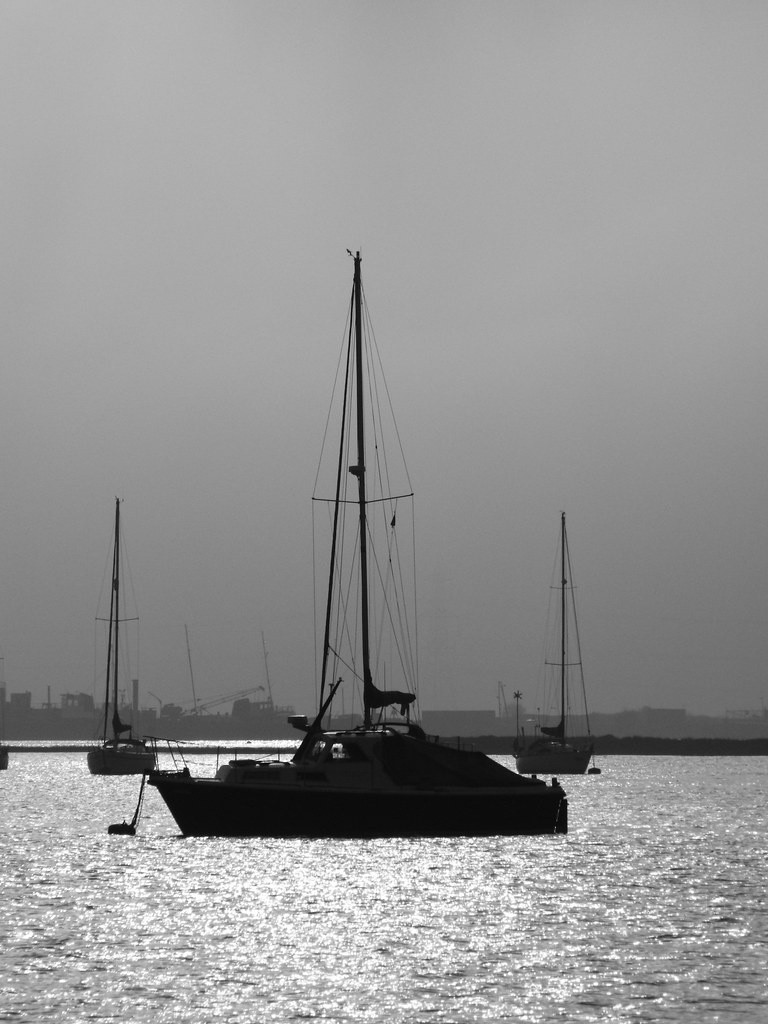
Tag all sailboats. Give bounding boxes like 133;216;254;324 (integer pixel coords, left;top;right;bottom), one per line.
87;499;157;776
146;248;570;839
513;510;595;774
